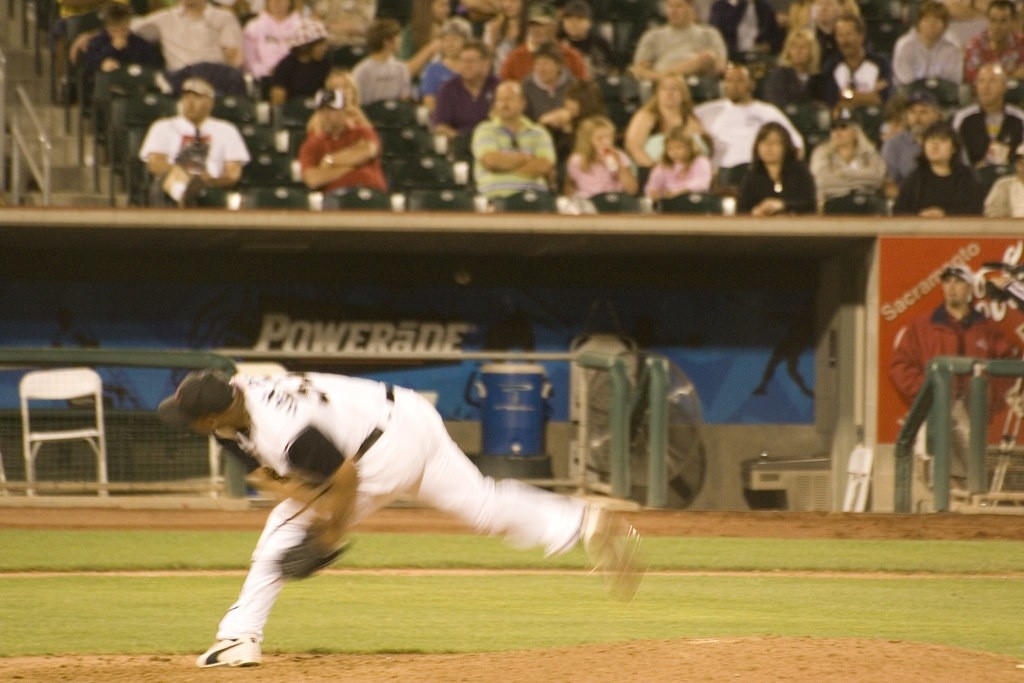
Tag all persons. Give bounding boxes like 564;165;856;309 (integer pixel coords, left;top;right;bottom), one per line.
39;0;1024;222
159;370;645;668
890;266;1024;496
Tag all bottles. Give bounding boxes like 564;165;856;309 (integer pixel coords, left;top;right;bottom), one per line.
603;150;618;172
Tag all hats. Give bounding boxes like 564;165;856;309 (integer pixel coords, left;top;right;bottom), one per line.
938;260;978;287
155;376;235;430
526;2;560;26
287;19;325;49
182;77;217;98
903;89;938;109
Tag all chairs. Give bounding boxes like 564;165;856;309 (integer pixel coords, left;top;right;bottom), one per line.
19;367;109;496
33;0;1024;216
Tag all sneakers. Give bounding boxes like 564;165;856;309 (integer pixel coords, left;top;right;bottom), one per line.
194;639;261;668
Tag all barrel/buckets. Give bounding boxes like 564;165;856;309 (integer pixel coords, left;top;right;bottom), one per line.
475;364;551;456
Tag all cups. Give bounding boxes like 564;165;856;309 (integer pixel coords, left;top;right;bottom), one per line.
417;106;428;123
640;198;652;213
328;90;341;108
392;193;404;211
257;103;270;124
308;192;322;210
474;195;485;213
433;134;447;153
227;193;240;209
724;198;736;216
452;161;468;184
292;161;300;182
275;130;289;150
